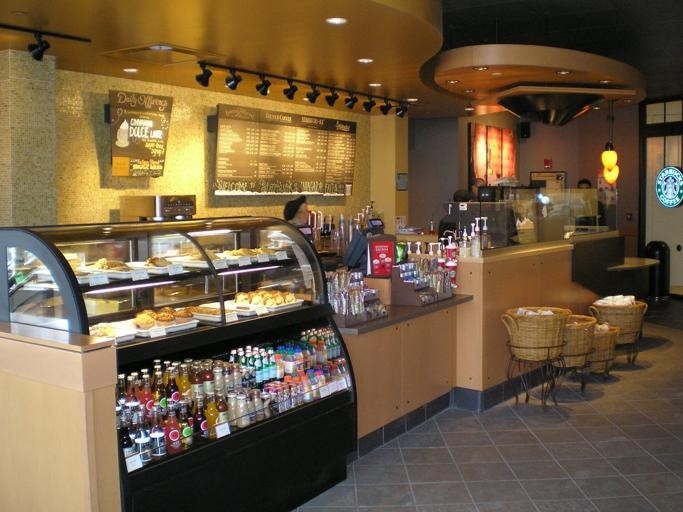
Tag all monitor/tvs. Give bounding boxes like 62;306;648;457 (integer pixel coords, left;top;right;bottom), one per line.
478;187;502;203
342;229;384;268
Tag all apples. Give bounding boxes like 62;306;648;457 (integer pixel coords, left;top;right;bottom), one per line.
182;427;192;437
160;398;167;408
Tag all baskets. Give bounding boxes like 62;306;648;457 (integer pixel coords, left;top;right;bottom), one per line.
587;296;647;345
500;304;570;361
589;323;619;375
553;312;596;368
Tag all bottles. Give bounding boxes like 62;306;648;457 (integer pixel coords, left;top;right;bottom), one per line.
305;204;371;258
323;271;365;314
116;326;350;471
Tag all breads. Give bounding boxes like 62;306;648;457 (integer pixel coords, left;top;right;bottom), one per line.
89;324;112;337
67;259;82;273
96;258;132;271
134;286;295;330
197;247;277;261
145;257;172;267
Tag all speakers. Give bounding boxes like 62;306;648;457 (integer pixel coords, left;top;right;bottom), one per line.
520;122;530;139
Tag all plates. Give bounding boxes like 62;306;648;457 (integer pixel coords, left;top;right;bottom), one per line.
31;246;281;284
90;294;302;342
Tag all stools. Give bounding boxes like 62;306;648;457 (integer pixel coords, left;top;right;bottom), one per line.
506;324;643;410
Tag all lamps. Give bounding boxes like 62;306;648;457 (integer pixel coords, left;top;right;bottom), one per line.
195;61;412;118
601;99;618;172
603;115;620;184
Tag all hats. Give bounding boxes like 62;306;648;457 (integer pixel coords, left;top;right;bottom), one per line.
282;196;306;221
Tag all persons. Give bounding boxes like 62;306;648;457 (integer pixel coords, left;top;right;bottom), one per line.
284;195;313;244
571;178;606;225
438;190;470;245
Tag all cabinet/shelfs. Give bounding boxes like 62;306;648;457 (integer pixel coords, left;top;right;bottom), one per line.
0;215;359;512
